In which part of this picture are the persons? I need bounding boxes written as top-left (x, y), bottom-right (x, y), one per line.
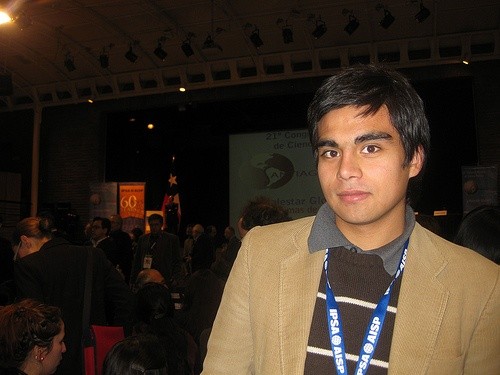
top-left (198, 62), bottom-right (500, 375)
top-left (0, 192), bottom-right (500, 375)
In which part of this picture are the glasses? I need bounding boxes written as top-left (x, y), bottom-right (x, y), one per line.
top-left (13, 241), bottom-right (22, 260)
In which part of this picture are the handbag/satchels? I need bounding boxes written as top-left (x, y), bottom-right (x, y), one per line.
top-left (82, 325), bottom-right (125, 375)
